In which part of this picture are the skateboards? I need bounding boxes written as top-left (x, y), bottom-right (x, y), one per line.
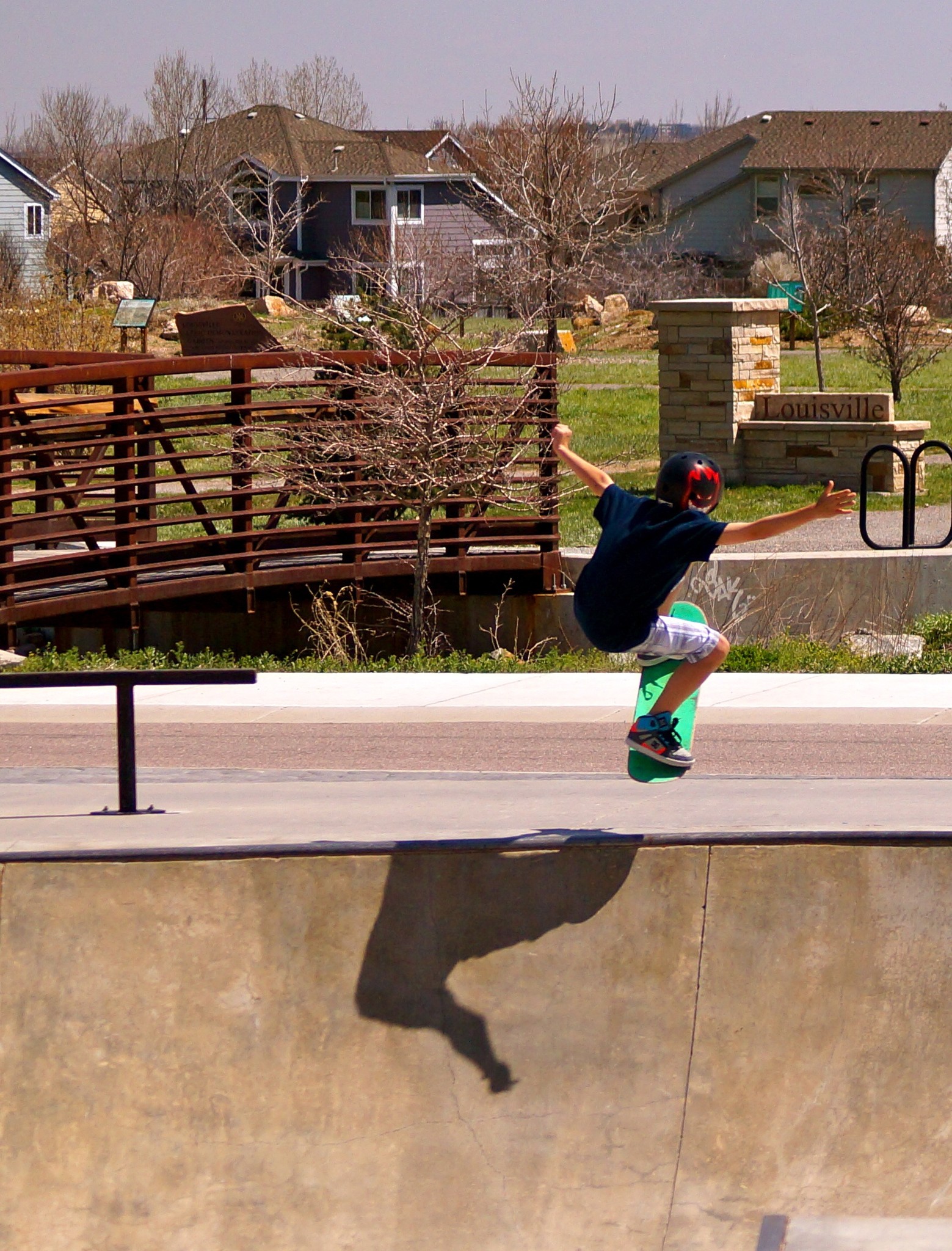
top-left (627, 601), bottom-right (708, 783)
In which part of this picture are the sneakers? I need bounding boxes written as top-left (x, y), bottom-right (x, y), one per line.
top-left (626, 711), bottom-right (697, 766)
top-left (636, 654), bottom-right (669, 666)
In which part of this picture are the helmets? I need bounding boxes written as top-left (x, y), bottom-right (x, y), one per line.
top-left (655, 451), bottom-right (723, 515)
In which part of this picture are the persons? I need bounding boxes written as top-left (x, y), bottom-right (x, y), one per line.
top-left (551, 423), bottom-right (857, 767)
